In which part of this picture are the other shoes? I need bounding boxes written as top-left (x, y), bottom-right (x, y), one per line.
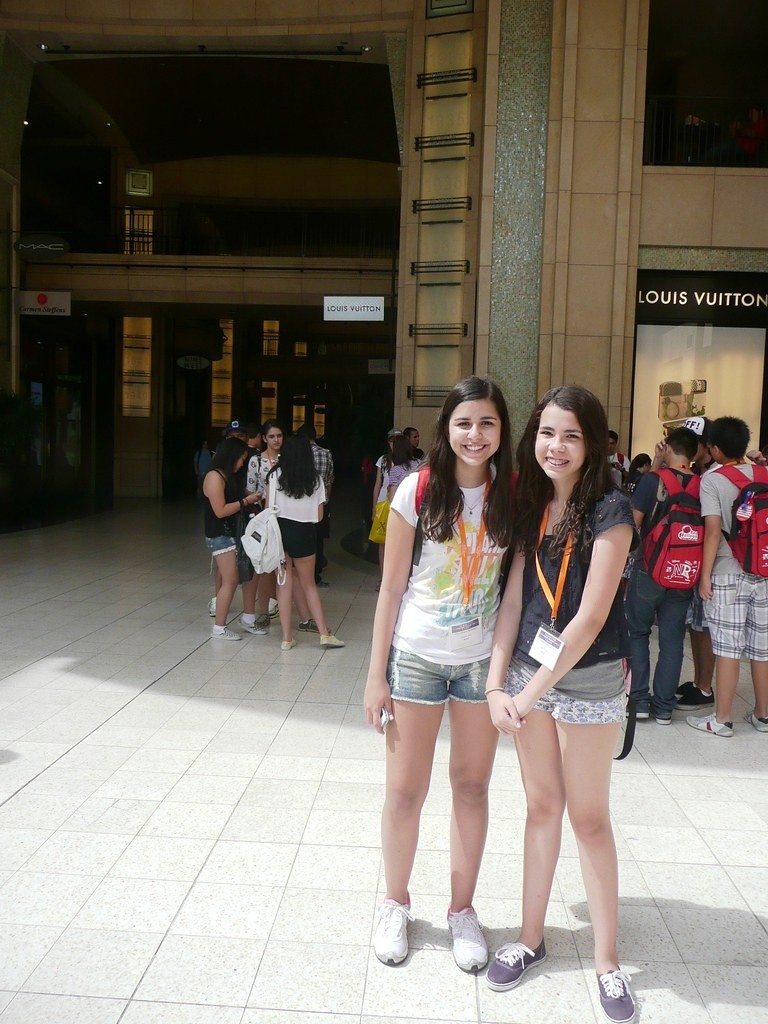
top-left (238, 615), bottom-right (268, 635)
top-left (257, 614), bottom-right (271, 626)
top-left (269, 607), bottom-right (279, 618)
top-left (210, 627), bottom-right (241, 640)
top-left (208, 600), bottom-right (217, 617)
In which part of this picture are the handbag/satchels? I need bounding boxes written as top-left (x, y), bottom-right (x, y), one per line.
top-left (236, 499), bottom-right (255, 583)
top-left (368, 500), bottom-right (390, 544)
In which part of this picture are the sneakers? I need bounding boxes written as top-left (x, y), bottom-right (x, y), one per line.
top-left (650, 707), bottom-right (671, 724)
top-left (374, 890), bottom-right (414, 965)
top-left (446, 900), bottom-right (488, 973)
top-left (674, 687), bottom-right (715, 710)
top-left (744, 710), bottom-right (768, 732)
top-left (626, 702), bottom-right (649, 718)
top-left (486, 936), bottom-right (546, 992)
top-left (298, 619), bottom-right (331, 633)
top-left (674, 681), bottom-right (696, 698)
top-left (319, 635), bottom-right (344, 648)
top-left (686, 713), bottom-right (734, 737)
top-left (281, 637), bottom-right (296, 651)
top-left (596, 964), bottom-right (637, 1024)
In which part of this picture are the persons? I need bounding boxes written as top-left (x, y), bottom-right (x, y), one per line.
top-left (403, 427), bottom-right (420, 447)
top-left (626, 427), bottom-right (698, 725)
top-left (674, 416), bottom-right (719, 711)
top-left (608, 431), bottom-right (631, 489)
top-left (369, 428), bottom-right (402, 592)
top-left (194, 419), bottom-right (286, 635)
top-left (362, 376), bottom-right (519, 971)
top-left (686, 416), bottom-right (768, 737)
top-left (265, 431), bottom-right (345, 651)
top-left (486, 385), bottom-right (639, 1024)
top-left (625, 453), bottom-right (652, 498)
top-left (389, 435), bottom-right (422, 502)
top-left (201, 437), bottom-right (263, 641)
top-left (296, 423), bottom-right (334, 587)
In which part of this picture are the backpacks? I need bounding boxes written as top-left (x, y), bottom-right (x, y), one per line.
top-left (709, 464), bottom-right (768, 577)
top-left (643, 469), bottom-right (703, 589)
top-left (241, 471), bottom-right (286, 586)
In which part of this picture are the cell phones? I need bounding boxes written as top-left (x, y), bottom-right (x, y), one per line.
top-left (380, 706), bottom-right (389, 729)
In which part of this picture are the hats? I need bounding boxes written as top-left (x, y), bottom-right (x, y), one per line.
top-left (226, 421), bottom-right (247, 433)
top-left (683, 417), bottom-right (713, 437)
top-left (388, 428), bottom-right (402, 439)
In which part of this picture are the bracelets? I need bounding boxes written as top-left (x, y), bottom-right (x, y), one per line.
top-left (245, 498), bottom-right (249, 506)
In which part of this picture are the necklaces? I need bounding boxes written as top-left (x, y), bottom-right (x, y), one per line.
top-left (461, 497), bottom-right (483, 515)
top-left (554, 498), bottom-right (568, 520)
